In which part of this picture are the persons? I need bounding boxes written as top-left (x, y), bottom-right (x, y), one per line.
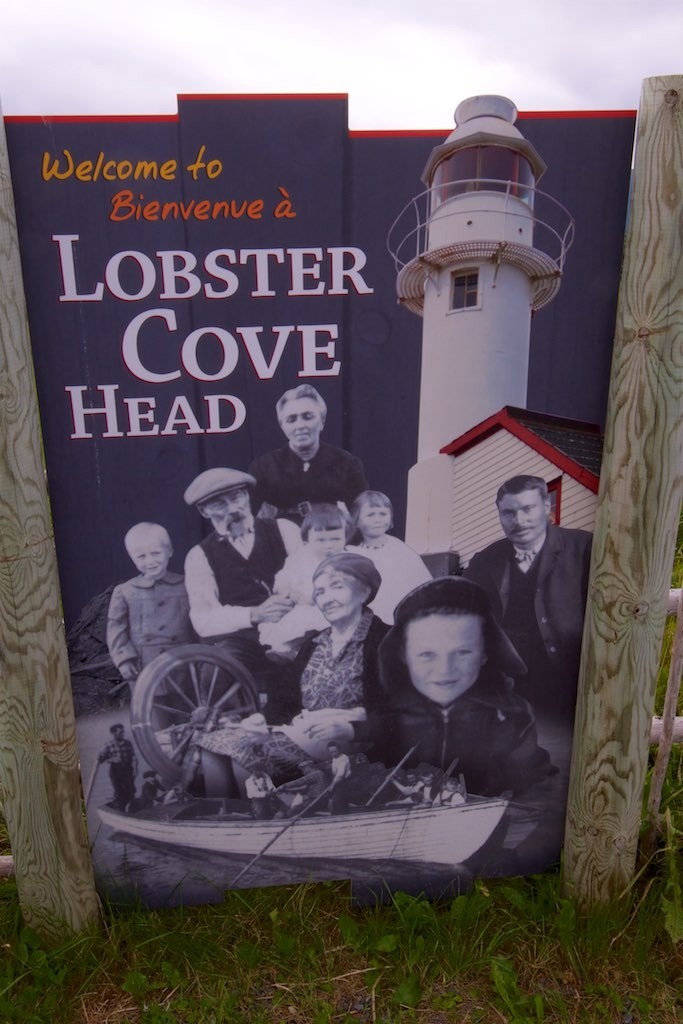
top-left (91, 382), bottom-right (595, 822)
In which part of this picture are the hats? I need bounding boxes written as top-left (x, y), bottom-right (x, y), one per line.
top-left (376, 575), bottom-right (528, 680)
top-left (182, 468), bottom-right (255, 507)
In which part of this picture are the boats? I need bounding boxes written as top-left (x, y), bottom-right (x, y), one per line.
top-left (94, 779), bottom-right (513, 869)
